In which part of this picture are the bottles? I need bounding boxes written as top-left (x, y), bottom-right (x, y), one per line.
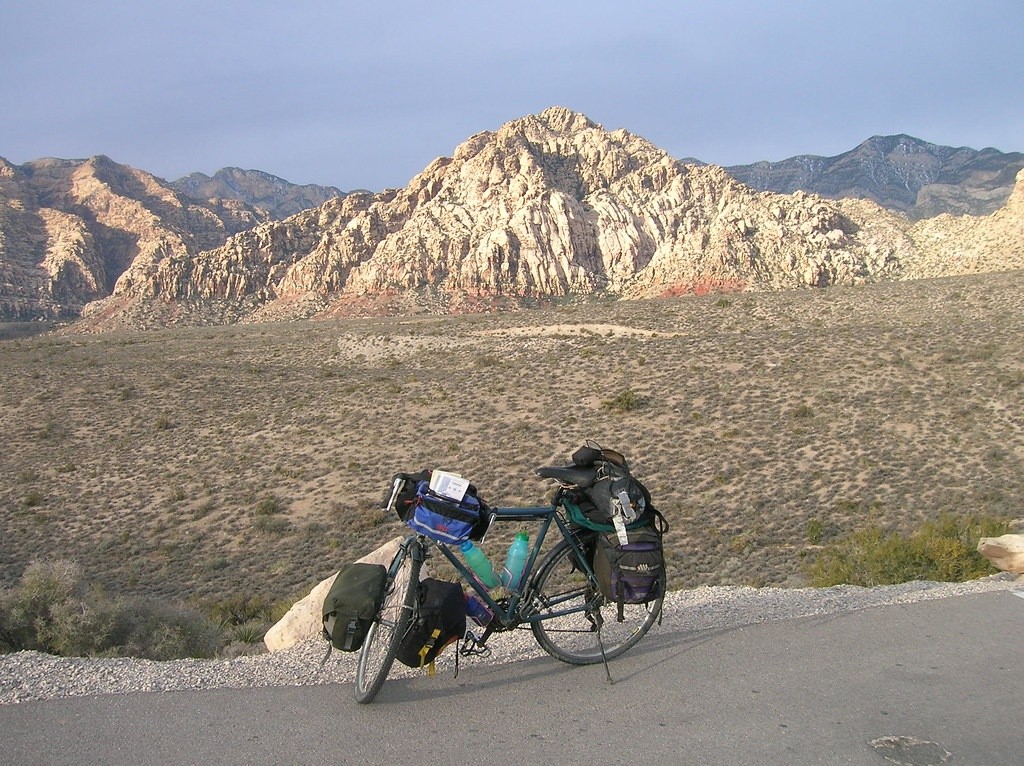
top-left (466, 596), bottom-right (493, 627)
top-left (460, 540), bottom-right (502, 589)
top-left (501, 530), bottom-right (529, 591)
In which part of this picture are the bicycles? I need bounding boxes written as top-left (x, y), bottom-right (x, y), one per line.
top-left (354, 463), bottom-right (667, 703)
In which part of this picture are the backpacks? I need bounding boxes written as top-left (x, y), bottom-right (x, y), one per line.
top-left (395, 577), bottom-right (466, 680)
top-left (319, 563), bottom-right (387, 664)
top-left (593, 526), bottom-right (666, 626)
top-left (562, 448), bottom-right (654, 533)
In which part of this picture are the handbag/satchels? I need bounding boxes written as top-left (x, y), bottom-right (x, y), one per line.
top-left (397, 469), bottom-right (497, 545)
